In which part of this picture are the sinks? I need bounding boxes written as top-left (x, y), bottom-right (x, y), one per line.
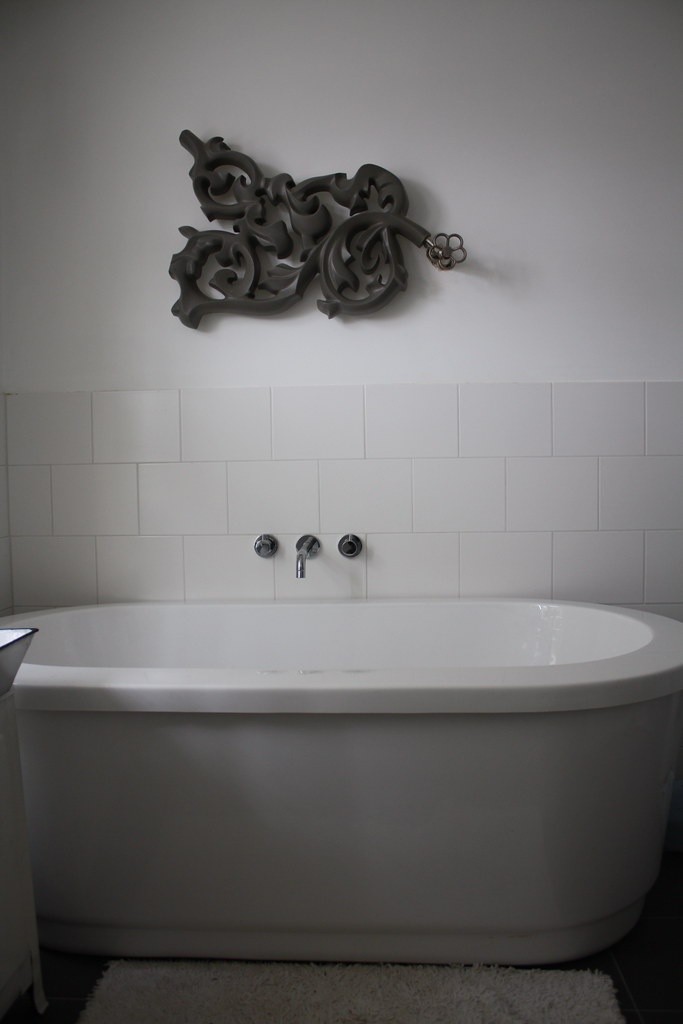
top-left (0, 629), bottom-right (39, 700)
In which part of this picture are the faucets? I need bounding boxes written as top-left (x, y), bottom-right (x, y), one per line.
top-left (296, 537), bottom-right (315, 579)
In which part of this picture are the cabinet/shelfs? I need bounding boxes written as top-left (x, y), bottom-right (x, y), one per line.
top-left (0, 691), bottom-right (48, 1015)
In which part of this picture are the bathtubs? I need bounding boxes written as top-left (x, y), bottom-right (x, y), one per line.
top-left (0, 602), bottom-right (682, 964)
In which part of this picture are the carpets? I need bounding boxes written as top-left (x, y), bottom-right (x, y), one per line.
top-left (80, 960), bottom-right (626, 1024)
